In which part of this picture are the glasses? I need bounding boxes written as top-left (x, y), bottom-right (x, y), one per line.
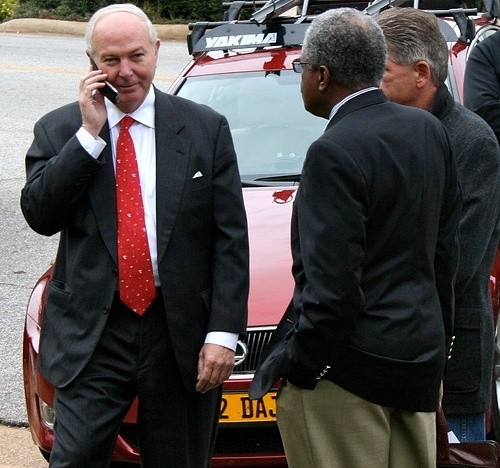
top-left (291, 58), bottom-right (311, 72)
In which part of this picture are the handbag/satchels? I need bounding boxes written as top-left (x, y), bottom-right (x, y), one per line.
top-left (438, 404), bottom-right (497, 468)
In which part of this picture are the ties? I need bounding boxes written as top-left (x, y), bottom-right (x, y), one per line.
top-left (114, 116), bottom-right (156, 317)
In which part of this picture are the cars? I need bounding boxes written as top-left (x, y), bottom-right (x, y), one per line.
top-left (21, 0), bottom-right (500, 468)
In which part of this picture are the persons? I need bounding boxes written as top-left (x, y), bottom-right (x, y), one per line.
top-left (247, 8), bottom-right (463, 468)
top-left (463, 28), bottom-right (500, 148)
top-left (371, 7), bottom-right (500, 442)
top-left (20, 3), bottom-right (249, 468)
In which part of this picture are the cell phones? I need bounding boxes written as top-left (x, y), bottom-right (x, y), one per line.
top-left (90, 58), bottom-right (118, 104)
